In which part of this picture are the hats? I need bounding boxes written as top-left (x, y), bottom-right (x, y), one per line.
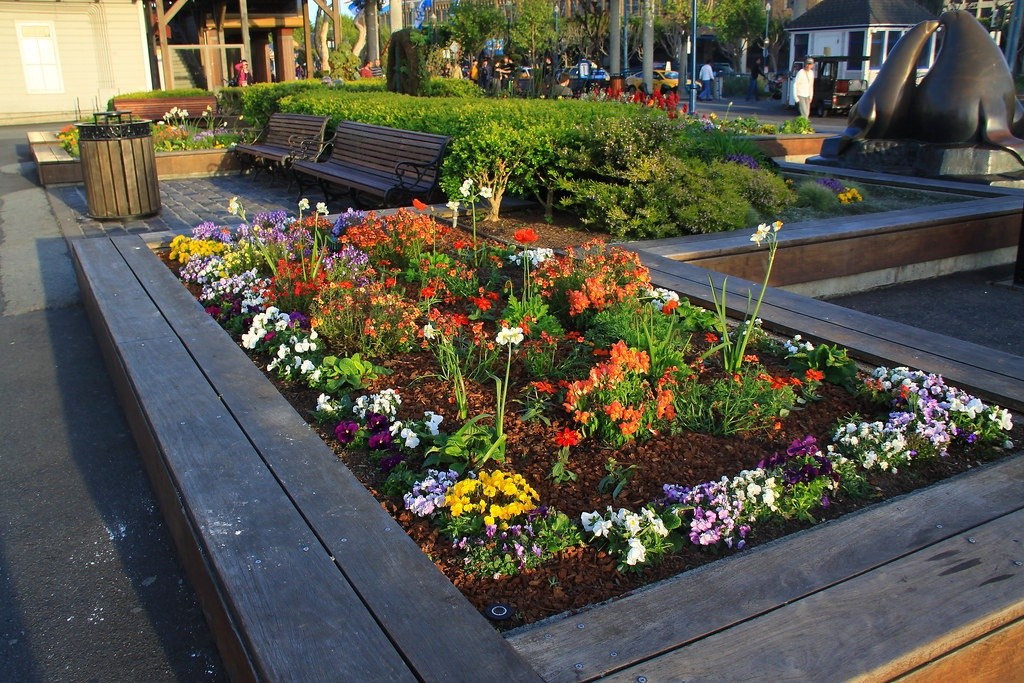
top-left (805, 58), bottom-right (814, 64)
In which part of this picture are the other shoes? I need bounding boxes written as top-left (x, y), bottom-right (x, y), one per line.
top-left (698, 96), bottom-right (702, 101)
top-left (706, 99), bottom-right (712, 101)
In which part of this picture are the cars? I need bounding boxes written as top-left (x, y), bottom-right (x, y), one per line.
top-left (623, 68), bottom-right (705, 101)
top-left (620, 61), bottom-right (790, 100)
top-left (459, 56), bottom-right (611, 99)
top-left (805, 54), bottom-right (873, 119)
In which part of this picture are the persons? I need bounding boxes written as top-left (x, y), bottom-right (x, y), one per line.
top-left (698, 60), bottom-right (714, 101)
top-left (492, 56), bottom-right (516, 94)
top-left (551, 76), bottom-right (573, 96)
top-left (793, 58), bottom-right (814, 119)
top-left (371, 59), bottom-right (382, 76)
top-left (296, 66), bottom-right (301, 77)
top-left (542, 58), bottom-right (554, 83)
top-left (362, 61), bottom-right (373, 78)
top-left (440, 61), bottom-right (462, 79)
top-left (235, 60), bottom-right (252, 86)
top-left (462, 64), bottom-right (468, 77)
top-left (477, 58), bottom-right (492, 92)
top-left (575, 56), bottom-right (597, 93)
top-left (469, 60), bottom-right (479, 80)
top-left (746, 58), bottom-right (766, 101)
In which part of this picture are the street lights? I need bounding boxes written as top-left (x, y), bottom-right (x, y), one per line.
top-left (553, 2), bottom-right (561, 62)
top-left (763, 3), bottom-right (772, 80)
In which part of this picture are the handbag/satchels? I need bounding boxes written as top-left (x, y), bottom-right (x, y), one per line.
top-left (230, 77), bottom-right (237, 86)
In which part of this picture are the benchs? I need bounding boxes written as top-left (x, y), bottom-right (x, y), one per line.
top-left (114, 96), bottom-right (227, 130)
top-left (234, 111), bottom-right (451, 211)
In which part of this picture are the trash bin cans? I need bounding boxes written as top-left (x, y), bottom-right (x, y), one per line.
top-left (74, 110), bottom-right (162, 219)
top-left (610, 76), bottom-right (625, 96)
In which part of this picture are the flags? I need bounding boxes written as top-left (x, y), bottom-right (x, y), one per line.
top-left (380, 3), bottom-right (390, 13)
top-left (415, 0), bottom-right (431, 29)
top-left (348, 2), bottom-right (360, 15)
